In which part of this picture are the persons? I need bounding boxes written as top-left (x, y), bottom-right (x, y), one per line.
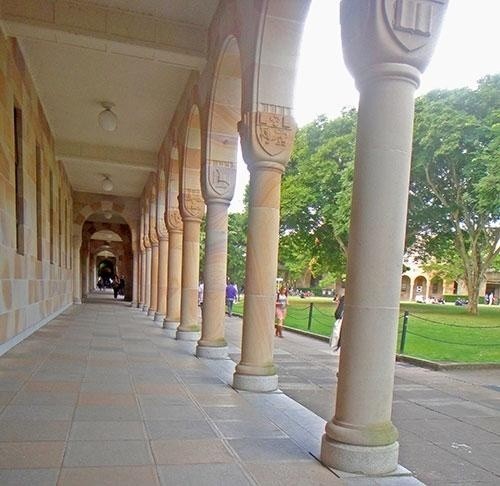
top-left (486, 292), bottom-right (495, 305)
top-left (97, 275), bottom-right (126, 300)
top-left (226, 281), bottom-right (237, 317)
top-left (288, 287), bottom-right (312, 298)
top-left (431, 297), bottom-right (469, 306)
top-left (198, 283), bottom-right (204, 316)
top-left (329, 296), bottom-right (345, 351)
top-left (275, 287), bottom-right (289, 339)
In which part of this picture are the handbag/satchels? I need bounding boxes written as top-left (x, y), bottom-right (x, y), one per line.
top-left (330, 319), bottom-right (343, 348)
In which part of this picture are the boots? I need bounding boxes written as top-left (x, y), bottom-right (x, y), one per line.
top-left (278, 326), bottom-right (284, 337)
top-left (276, 326), bottom-right (278, 336)
